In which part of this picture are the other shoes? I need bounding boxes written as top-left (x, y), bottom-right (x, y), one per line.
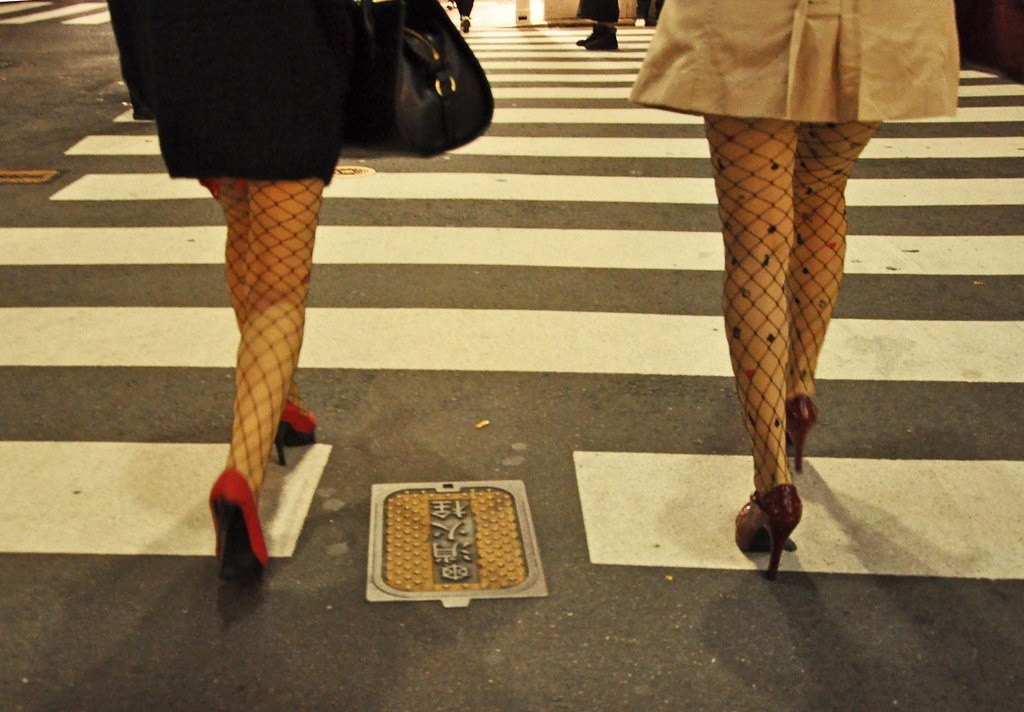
top-left (576, 24), bottom-right (618, 50)
top-left (461, 16), bottom-right (470, 33)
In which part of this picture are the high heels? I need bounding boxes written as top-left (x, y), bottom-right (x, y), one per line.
top-left (275, 399), bottom-right (318, 466)
top-left (735, 484), bottom-right (803, 578)
top-left (208, 469), bottom-right (270, 591)
top-left (786, 393), bottom-right (820, 475)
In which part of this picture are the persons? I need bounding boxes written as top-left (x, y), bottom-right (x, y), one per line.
top-left (575, 0), bottom-right (620, 50)
top-left (630, 0), bottom-right (960, 579)
top-left (446, 0), bottom-right (474, 33)
top-left (635, 0), bottom-right (665, 27)
top-left (108, 0), bottom-right (367, 584)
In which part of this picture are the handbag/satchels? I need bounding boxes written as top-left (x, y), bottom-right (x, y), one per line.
top-left (360, 0), bottom-right (494, 156)
